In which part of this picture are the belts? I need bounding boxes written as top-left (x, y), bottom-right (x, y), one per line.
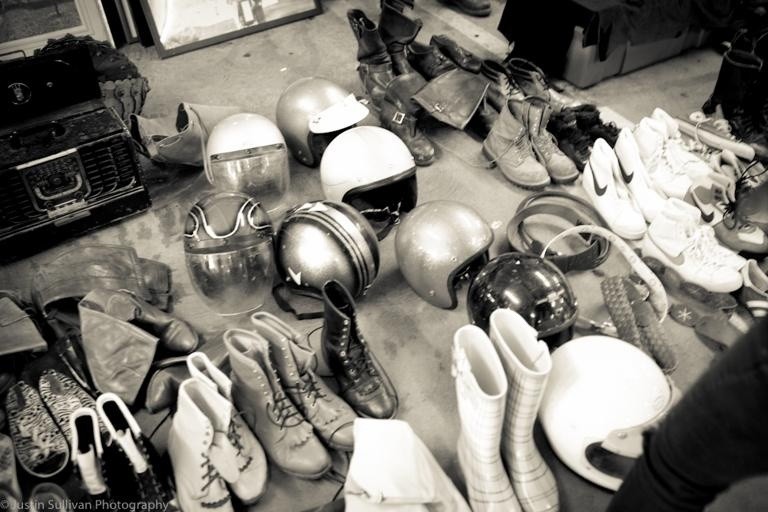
top-left (506, 190), bottom-right (612, 271)
top-left (601, 276), bottom-right (680, 374)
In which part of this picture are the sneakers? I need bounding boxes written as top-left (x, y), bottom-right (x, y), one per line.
top-left (580, 107), bottom-right (768, 323)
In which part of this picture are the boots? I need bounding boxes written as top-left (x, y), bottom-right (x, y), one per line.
top-left (450, 324), bottom-right (523, 512)
top-left (0, 245), bottom-right (398, 512)
top-left (343, 416), bottom-right (471, 510)
top-left (701, 50), bottom-right (767, 147)
top-left (346, 0), bottom-right (621, 192)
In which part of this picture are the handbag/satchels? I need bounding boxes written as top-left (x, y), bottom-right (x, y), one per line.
top-left (128, 100), bottom-right (240, 170)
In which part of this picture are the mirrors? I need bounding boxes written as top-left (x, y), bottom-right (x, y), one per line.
top-left (138, 0), bottom-right (325, 61)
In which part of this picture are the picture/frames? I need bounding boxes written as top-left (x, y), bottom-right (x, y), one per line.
top-left (0, 0), bottom-right (117, 62)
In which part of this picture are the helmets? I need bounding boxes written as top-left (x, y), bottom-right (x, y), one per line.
top-left (538, 336), bottom-right (683, 496)
top-left (488, 308), bottom-right (561, 512)
top-left (183, 76), bottom-right (419, 318)
top-left (394, 198), bottom-right (494, 312)
top-left (468, 252), bottom-right (578, 347)
